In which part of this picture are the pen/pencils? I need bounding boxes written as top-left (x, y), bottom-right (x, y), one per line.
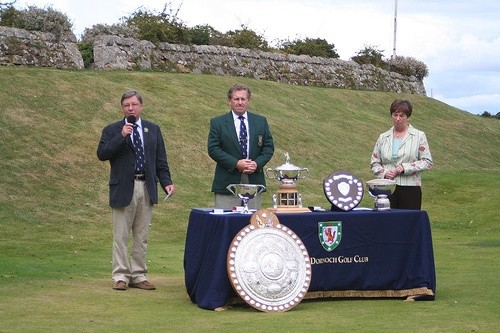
top-left (164, 194), bottom-right (169, 201)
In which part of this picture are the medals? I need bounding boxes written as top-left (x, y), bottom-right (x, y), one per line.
top-left (227, 210), bottom-right (312, 313)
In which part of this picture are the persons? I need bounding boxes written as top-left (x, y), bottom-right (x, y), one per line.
top-left (96, 91), bottom-right (175, 291)
top-left (369, 99), bottom-right (434, 210)
top-left (206, 83), bottom-right (276, 210)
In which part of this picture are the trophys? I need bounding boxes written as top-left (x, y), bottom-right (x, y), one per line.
top-left (323, 168), bottom-right (364, 212)
top-left (365, 179), bottom-right (398, 211)
top-left (226, 183), bottom-right (267, 214)
top-left (265, 152), bottom-right (311, 213)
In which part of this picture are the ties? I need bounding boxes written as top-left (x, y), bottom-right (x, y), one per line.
top-left (237, 115), bottom-right (247, 161)
top-left (132, 124), bottom-right (145, 173)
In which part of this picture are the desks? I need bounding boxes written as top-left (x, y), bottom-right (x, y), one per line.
top-left (184, 208), bottom-right (436, 311)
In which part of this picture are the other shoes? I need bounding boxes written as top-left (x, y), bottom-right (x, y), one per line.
top-left (111, 281), bottom-right (129, 289)
top-left (130, 280), bottom-right (157, 289)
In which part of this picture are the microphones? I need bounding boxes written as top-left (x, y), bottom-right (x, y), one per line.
top-left (125, 115), bottom-right (136, 145)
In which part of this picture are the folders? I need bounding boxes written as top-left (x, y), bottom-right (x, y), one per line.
top-left (191, 208), bottom-right (233, 213)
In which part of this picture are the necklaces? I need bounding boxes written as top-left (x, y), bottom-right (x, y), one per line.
top-left (394, 129), bottom-right (407, 137)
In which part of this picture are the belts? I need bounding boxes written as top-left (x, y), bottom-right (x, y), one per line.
top-left (134, 176), bottom-right (144, 181)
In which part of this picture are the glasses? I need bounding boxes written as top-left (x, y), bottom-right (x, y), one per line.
top-left (122, 102), bottom-right (140, 108)
